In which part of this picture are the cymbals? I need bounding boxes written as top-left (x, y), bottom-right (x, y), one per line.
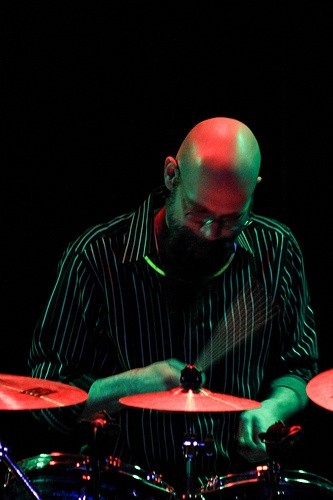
top-left (0, 371), bottom-right (89, 412)
top-left (305, 368), bottom-right (333, 413)
top-left (118, 365), bottom-right (263, 413)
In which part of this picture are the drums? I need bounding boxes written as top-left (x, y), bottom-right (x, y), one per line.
top-left (0, 450), bottom-right (174, 498)
top-left (193, 461), bottom-right (333, 499)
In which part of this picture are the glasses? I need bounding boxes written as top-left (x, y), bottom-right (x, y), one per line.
top-left (178, 167), bottom-right (255, 232)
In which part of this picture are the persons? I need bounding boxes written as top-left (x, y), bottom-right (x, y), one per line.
top-left (27, 117), bottom-right (319, 464)
